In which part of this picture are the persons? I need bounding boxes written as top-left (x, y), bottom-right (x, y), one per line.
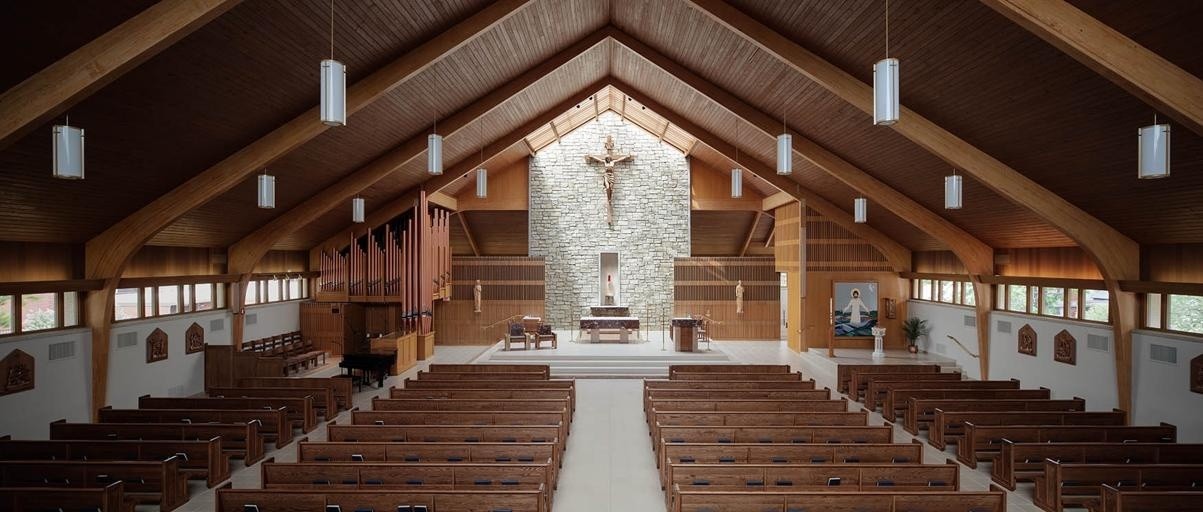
top-left (842, 288), bottom-right (871, 324)
top-left (735, 279), bottom-right (745, 312)
top-left (589, 155), bottom-right (631, 203)
top-left (474, 280), bottom-right (481, 311)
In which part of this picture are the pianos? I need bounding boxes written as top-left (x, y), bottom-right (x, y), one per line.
top-left (339, 347), bottom-right (398, 391)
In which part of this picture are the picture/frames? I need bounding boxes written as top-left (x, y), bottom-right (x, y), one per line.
top-left (832, 278), bottom-right (880, 340)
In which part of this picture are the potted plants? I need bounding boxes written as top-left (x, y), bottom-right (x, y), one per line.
top-left (903, 316), bottom-right (929, 353)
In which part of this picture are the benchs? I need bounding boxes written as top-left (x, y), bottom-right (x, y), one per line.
top-left (1, 329), bottom-right (573, 511)
top-left (642, 361), bottom-right (1203, 509)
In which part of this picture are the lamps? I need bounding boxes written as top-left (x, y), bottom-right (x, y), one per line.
top-left (427, 63), bottom-right (443, 174)
top-left (321, 0), bottom-right (348, 127)
top-left (352, 194), bottom-right (366, 223)
top-left (732, 116), bottom-right (744, 199)
top-left (777, 61), bottom-right (793, 176)
top-left (945, 165), bottom-right (962, 209)
top-left (855, 194), bottom-right (867, 223)
top-left (477, 118), bottom-right (488, 197)
top-left (872, 0), bottom-right (901, 126)
top-left (52, 113), bottom-right (86, 181)
top-left (1137, 115), bottom-right (1177, 181)
top-left (258, 168), bottom-right (277, 208)
